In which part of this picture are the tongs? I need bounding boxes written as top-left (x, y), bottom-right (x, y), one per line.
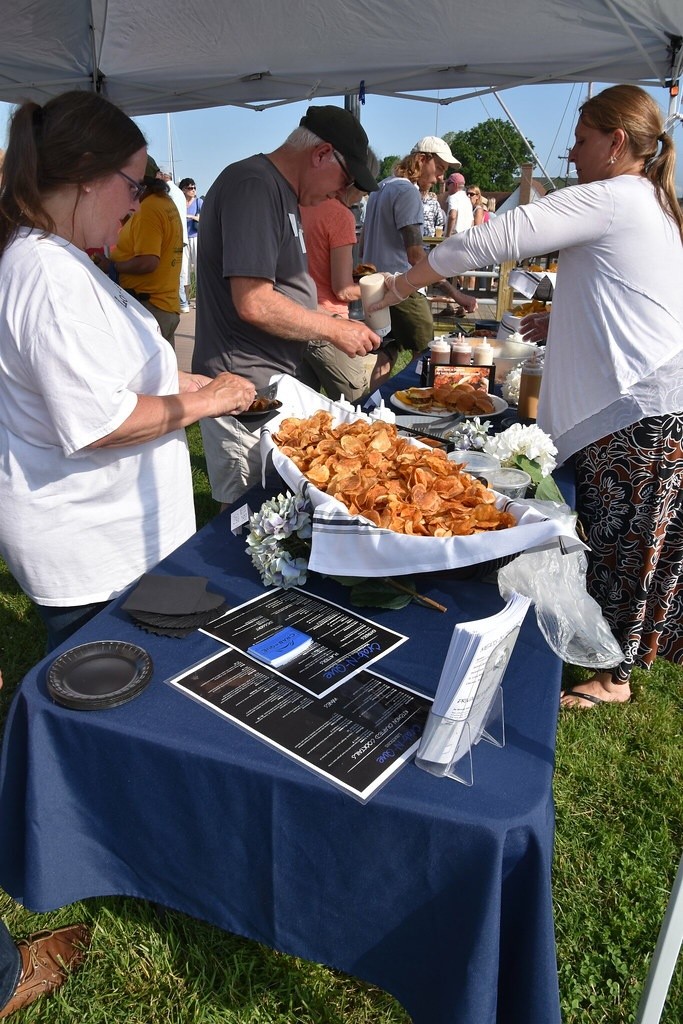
top-left (412, 413), bottom-right (464, 438)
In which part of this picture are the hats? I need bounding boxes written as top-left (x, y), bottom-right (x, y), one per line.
top-left (301, 106), bottom-right (380, 193)
top-left (444, 173), bottom-right (465, 185)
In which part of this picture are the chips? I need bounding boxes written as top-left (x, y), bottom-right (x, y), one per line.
top-left (272, 409), bottom-right (517, 537)
top-left (513, 299), bottom-right (552, 315)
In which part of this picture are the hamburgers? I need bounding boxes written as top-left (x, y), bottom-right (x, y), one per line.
top-left (396, 382), bottom-right (495, 415)
top-left (359, 261), bottom-right (376, 275)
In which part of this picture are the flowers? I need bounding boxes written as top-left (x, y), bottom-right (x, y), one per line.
top-left (449, 418), bottom-right (589, 539)
top-left (244, 491), bottom-right (447, 610)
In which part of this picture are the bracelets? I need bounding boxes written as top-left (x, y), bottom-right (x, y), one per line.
top-left (405, 272), bottom-right (419, 290)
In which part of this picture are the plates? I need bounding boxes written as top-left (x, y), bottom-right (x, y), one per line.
top-left (48, 640), bottom-right (154, 711)
top-left (234, 399), bottom-right (282, 417)
top-left (389, 387), bottom-right (508, 418)
top-left (392, 416), bottom-right (466, 451)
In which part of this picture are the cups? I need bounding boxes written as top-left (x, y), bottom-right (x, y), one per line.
top-left (435, 225), bottom-right (444, 237)
top-left (446, 450), bottom-right (530, 499)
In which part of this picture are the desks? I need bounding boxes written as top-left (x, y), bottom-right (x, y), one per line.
top-left (0, 349), bottom-right (573, 1024)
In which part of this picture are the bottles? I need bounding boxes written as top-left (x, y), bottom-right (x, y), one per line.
top-left (430, 332), bottom-right (493, 368)
top-left (334, 392), bottom-right (395, 425)
top-left (523, 250), bottom-right (559, 271)
top-left (359, 273), bottom-right (392, 337)
top-left (518, 351), bottom-right (543, 419)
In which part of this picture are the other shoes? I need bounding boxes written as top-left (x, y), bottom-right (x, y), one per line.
top-left (180, 307), bottom-right (189, 312)
top-left (0, 922), bottom-right (90, 1018)
top-left (454, 309), bottom-right (467, 316)
top-left (434, 308), bottom-right (454, 317)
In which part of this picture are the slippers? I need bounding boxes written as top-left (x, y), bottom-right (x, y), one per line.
top-left (559, 685), bottom-right (635, 713)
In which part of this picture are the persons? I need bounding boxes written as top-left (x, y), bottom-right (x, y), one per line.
top-left (0, 91), bottom-right (255, 648)
top-left (420, 172), bottom-right (497, 317)
top-left (299, 145), bottom-right (380, 406)
top-left (0, 918), bottom-right (91, 1019)
top-left (190, 105), bottom-right (382, 511)
top-left (368, 85), bottom-right (683, 708)
top-left (156, 169), bottom-right (189, 314)
top-left (358, 136), bottom-right (479, 394)
top-left (93, 154), bottom-right (183, 348)
top-left (178, 178), bottom-right (203, 308)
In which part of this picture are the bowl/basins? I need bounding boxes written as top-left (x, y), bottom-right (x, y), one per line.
top-left (475, 319), bottom-right (499, 332)
top-left (427, 337), bottom-right (545, 378)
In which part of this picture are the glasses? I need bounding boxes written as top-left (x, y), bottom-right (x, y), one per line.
top-left (185, 187), bottom-right (195, 191)
top-left (96, 154), bottom-right (148, 202)
top-left (447, 181), bottom-right (454, 185)
top-left (410, 136), bottom-right (462, 170)
top-left (313, 143), bottom-right (355, 189)
top-left (466, 192), bottom-right (478, 197)
